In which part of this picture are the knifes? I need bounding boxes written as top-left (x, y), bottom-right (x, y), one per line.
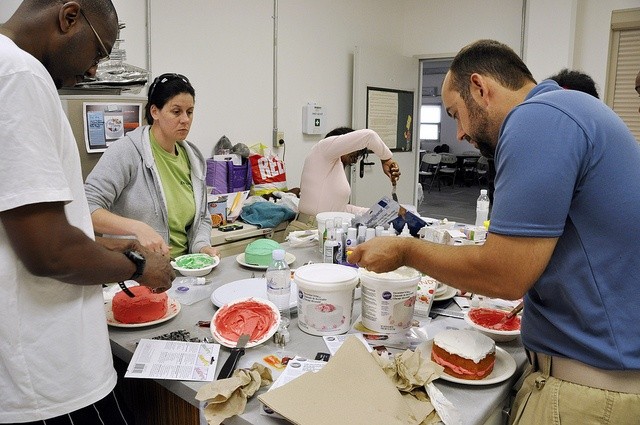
top-left (216, 314), bottom-right (261, 386)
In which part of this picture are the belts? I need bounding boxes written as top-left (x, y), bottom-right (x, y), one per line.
top-left (525, 347), bottom-right (640, 395)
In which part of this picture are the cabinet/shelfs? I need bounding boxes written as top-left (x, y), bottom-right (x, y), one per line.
top-left (457, 151), bottom-right (480, 185)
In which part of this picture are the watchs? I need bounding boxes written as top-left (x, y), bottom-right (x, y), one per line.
top-left (118, 249), bottom-right (146, 298)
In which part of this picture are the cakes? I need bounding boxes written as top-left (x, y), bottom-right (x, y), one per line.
top-left (244, 239), bottom-right (281, 267)
top-left (431, 329), bottom-right (496, 381)
top-left (111, 285), bottom-right (169, 324)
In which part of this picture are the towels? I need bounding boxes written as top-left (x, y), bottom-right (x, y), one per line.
top-left (286, 229), bottom-right (318, 245)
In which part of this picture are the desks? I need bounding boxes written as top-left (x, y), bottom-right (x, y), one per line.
top-left (456, 155), bottom-right (481, 188)
top-left (105, 241), bottom-right (530, 425)
top-left (211, 223), bottom-right (288, 257)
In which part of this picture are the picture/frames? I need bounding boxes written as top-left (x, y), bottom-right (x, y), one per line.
top-left (366, 86), bottom-right (414, 152)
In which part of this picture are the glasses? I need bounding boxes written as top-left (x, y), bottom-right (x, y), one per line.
top-left (150, 72), bottom-right (189, 101)
top-left (62, 0), bottom-right (110, 64)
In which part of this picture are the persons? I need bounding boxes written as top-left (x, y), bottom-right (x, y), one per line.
top-left (283, 126), bottom-right (401, 242)
top-left (0, 0), bottom-right (177, 425)
top-left (345, 38), bottom-right (640, 425)
top-left (549, 67), bottom-right (600, 100)
top-left (83, 72), bottom-right (221, 263)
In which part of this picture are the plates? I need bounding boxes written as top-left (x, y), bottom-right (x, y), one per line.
top-left (236, 251), bottom-right (299, 268)
top-left (208, 297), bottom-right (282, 350)
top-left (208, 276), bottom-right (315, 312)
top-left (101, 285), bottom-right (180, 328)
top-left (414, 336), bottom-right (518, 387)
top-left (433, 280), bottom-right (459, 303)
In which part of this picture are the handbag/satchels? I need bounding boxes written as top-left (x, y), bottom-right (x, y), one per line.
top-left (206, 156), bottom-right (252, 194)
top-left (247, 142), bottom-right (287, 197)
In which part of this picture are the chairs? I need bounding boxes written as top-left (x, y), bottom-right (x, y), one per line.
top-left (439, 153), bottom-right (458, 191)
top-left (419, 154), bottom-right (441, 194)
top-left (478, 157), bottom-right (489, 189)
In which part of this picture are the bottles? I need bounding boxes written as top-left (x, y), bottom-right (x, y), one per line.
top-left (323, 216), bottom-right (415, 266)
top-left (265, 247), bottom-right (292, 330)
top-left (474, 188), bottom-right (490, 242)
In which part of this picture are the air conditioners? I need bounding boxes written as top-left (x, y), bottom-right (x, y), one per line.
top-left (422, 87), bottom-right (437, 98)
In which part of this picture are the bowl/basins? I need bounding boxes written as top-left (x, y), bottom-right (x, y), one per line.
top-left (171, 253), bottom-right (221, 278)
top-left (465, 306), bottom-right (526, 343)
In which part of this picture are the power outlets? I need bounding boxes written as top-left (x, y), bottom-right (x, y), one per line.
top-left (274, 129), bottom-right (284, 148)
